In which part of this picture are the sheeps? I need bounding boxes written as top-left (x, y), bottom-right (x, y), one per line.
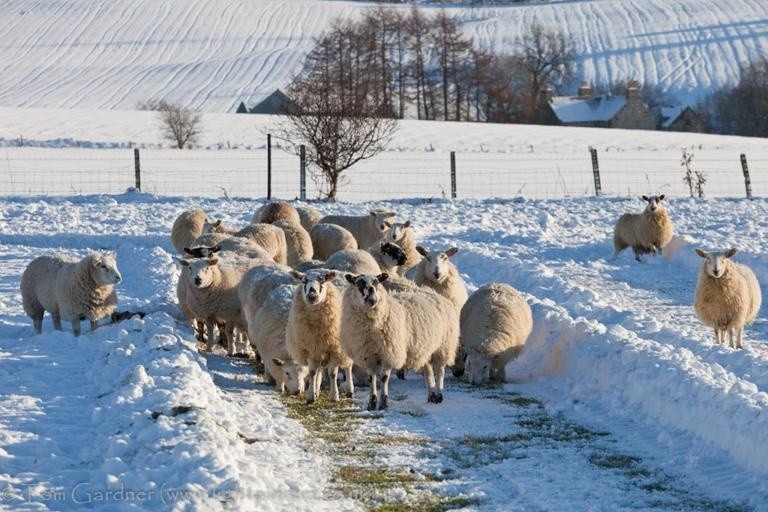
top-left (614, 194), bottom-right (673, 262)
top-left (171, 199), bottom-right (468, 412)
top-left (693, 247), bottom-right (762, 349)
top-left (20, 250), bottom-right (123, 338)
top-left (460, 282), bottom-right (533, 386)
